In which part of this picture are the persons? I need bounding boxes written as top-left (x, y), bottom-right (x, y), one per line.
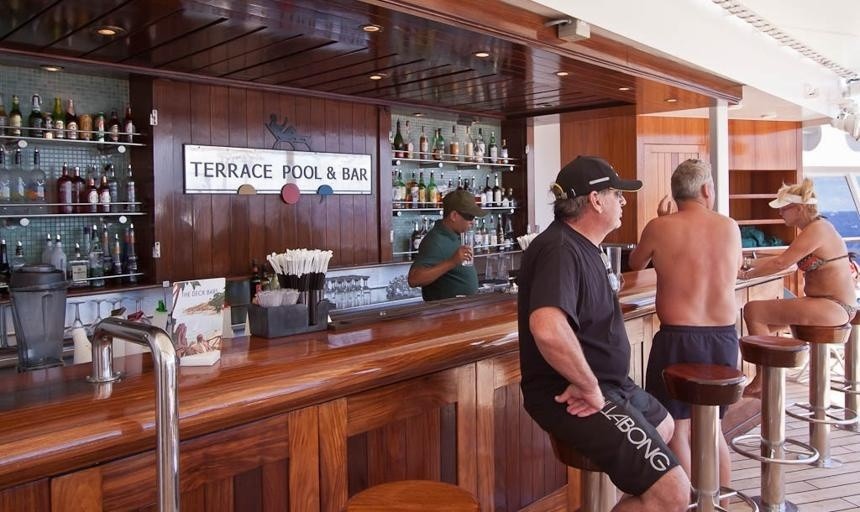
top-left (518, 155), bottom-right (692, 512)
top-left (408, 189), bottom-right (488, 301)
top-left (629, 159), bottom-right (742, 512)
top-left (737, 177), bottom-right (857, 394)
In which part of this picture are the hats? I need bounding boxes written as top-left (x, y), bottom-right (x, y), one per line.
top-left (552, 157), bottom-right (642, 193)
top-left (443, 190), bottom-right (488, 217)
top-left (768, 191), bottom-right (817, 209)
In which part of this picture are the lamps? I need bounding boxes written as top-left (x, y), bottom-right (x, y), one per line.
top-left (359, 22), bottom-right (384, 34)
top-left (95, 25), bottom-right (125, 40)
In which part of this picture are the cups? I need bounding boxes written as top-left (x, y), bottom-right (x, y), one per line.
top-left (325, 275), bottom-right (370, 299)
top-left (108, 299), bottom-right (118, 310)
top-left (117, 297), bottom-right (128, 308)
top-left (461, 232), bottom-right (473, 266)
top-left (132, 298), bottom-right (140, 312)
top-left (90, 300), bottom-right (105, 327)
top-left (607, 246), bottom-right (621, 292)
top-left (68, 302), bottom-right (86, 327)
top-left (138, 295), bottom-right (146, 310)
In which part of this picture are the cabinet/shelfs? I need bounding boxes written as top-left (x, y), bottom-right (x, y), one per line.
top-left (729, 119), bottom-right (804, 247)
top-left (625, 315), bottom-right (654, 391)
top-left (50, 406), bottom-right (320, 512)
top-left (478, 352), bottom-right (581, 511)
top-left (0, 125), bottom-right (150, 286)
top-left (749, 279), bottom-right (787, 430)
top-left (561, 116), bottom-right (710, 245)
top-left (319, 363), bottom-right (478, 512)
top-left (733, 288), bottom-right (749, 437)
top-left (392, 147), bottom-right (523, 256)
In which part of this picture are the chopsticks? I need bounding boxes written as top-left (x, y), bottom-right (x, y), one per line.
top-left (268, 249), bottom-right (333, 291)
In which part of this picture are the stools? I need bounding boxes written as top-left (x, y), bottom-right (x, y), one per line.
top-left (551, 436), bottom-right (619, 512)
top-left (791, 321), bottom-right (852, 469)
top-left (831, 311), bottom-right (860, 432)
top-left (342, 479), bottom-right (479, 511)
top-left (662, 363), bottom-right (761, 511)
top-left (739, 336), bottom-right (812, 511)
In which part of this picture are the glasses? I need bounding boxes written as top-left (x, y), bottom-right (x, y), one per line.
top-left (456, 211), bottom-right (475, 220)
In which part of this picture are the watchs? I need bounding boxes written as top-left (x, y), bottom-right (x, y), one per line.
top-left (747, 257), bottom-right (754, 269)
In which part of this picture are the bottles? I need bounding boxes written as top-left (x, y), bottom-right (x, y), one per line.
top-left (29, 147), bottom-right (48, 214)
top-left (0, 150), bottom-right (13, 216)
top-left (475, 127), bottom-right (486, 165)
top-left (93, 112), bottom-right (108, 141)
top-left (474, 217), bottom-right (483, 256)
top-left (474, 186), bottom-right (486, 208)
top-left (107, 164), bottom-right (123, 213)
top-left (65, 99), bottom-right (80, 139)
top-left (464, 178), bottom-right (471, 191)
top-left (28, 93), bottom-right (44, 138)
top-left (464, 126), bottom-right (474, 162)
top-left (1, 92), bottom-right (9, 136)
top-left (481, 218), bottom-right (490, 254)
top-left (408, 171), bottom-right (419, 210)
top-left (6, 95), bottom-right (23, 137)
top-left (394, 119), bottom-right (406, 159)
top-left (412, 221), bottom-right (422, 260)
top-left (83, 177), bottom-right (99, 213)
top-left (392, 168), bottom-right (402, 209)
top-left (40, 233), bottom-right (55, 265)
top-left (57, 161), bottom-right (74, 214)
top-left (122, 164), bottom-right (140, 212)
top-left (68, 243), bottom-right (90, 289)
top-left (448, 178), bottom-right (455, 193)
top-left (108, 233), bottom-right (125, 286)
top-left (98, 225), bottom-right (115, 278)
top-left (106, 110), bottom-right (123, 141)
top-left (495, 214), bottom-right (505, 252)
top-left (42, 111), bottom-right (55, 138)
top-left (488, 131), bottom-right (499, 164)
top-left (10, 242), bottom-right (29, 274)
top-left (488, 215), bottom-right (498, 253)
top-left (418, 172), bottom-right (427, 208)
top-left (500, 140), bottom-right (510, 164)
top-left (422, 217), bottom-right (429, 238)
top-left (121, 102), bottom-right (136, 143)
top-left (79, 114), bottom-right (94, 141)
top-left (507, 188), bottom-right (518, 207)
top-left (250, 258), bottom-right (262, 302)
top-left (493, 173), bottom-right (502, 207)
top-left (467, 177), bottom-right (477, 195)
top-left (122, 223), bottom-right (140, 284)
top-left (449, 125), bottom-right (460, 162)
top-left (72, 166), bottom-right (86, 214)
top-left (261, 264), bottom-right (272, 293)
top-left (456, 176), bottom-right (464, 190)
top-left (436, 129), bottom-right (445, 159)
top-left (399, 169), bottom-right (407, 209)
top-left (98, 176), bottom-right (112, 213)
top-left (50, 234), bottom-right (67, 282)
top-left (406, 122), bottom-right (414, 160)
top-left (431, 130), bottom-right (438, 157)
top-left (82, 227), bottom-right (91, 256)
top-left (53, 96), bottom-right (67, 140)
top-left (86, 224), bottom-right (106, 288)
top-left (427, 170), bottom-right (438, 208)
top-left (501, 187), bottom-right (510, 206)
top-left (9, 148), bottom-right (30, 215)
top-left (123, 227), bottom-right (130, 270)
top-left (271, 271), bottom-right (280, 290)
top-left (1, 238), bottom-right (11, 297)
top-left (419, 126), bottom-right (428, 160)
top-left (507, 219), bottom-right (515, 251)
top-left (437, 172), bottom-right (448, 208)
top-left (483, 174), bottom-right (494, 209)
top-left (503, 228), bottom-right (510, 252)
